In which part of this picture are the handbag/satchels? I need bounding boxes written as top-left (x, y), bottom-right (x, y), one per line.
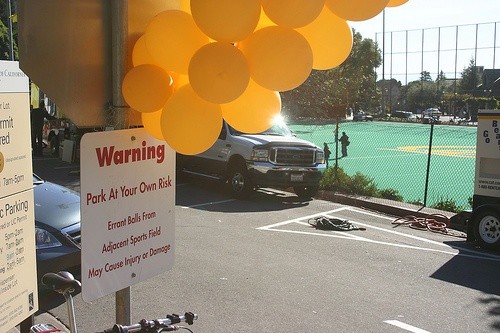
top-left (346, 141), bottom-right (350, 146)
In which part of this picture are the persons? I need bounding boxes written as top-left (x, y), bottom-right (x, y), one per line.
top-left (31, 103), bottom-right (55, 155)
top-left (339, 132), bottom-right (349, 157)
top-left (324, 143), bottom-right (330, 163)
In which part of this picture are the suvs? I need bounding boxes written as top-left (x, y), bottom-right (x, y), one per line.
top-left (176, 114), bottom-right (327, 201)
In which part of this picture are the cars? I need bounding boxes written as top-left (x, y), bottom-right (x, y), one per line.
top-left (354, 109), bottom-right (374, 122)
top-left (33, 173), bottom-right (81, 281)
top-left (422, 107), bottom-right (442, 119)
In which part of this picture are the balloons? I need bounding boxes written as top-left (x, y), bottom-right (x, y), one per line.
top-left (160, 84), bottom-right (222, 155)
top-left (142, 108), bottom-right (164, 140)
top-left (221, 79), bottom-right (281, 133)
top-left (145, 10), bottom-right (208, 75)
top-left (189, 42), bottom-right (250, 103)
top-left (254, 0), bottom-right (409, 70)
top-left (132, 35), bottom-right (188, 88)
top-left (190, 0), bottom-right (261, 42)
top-left (122, 64), bottom-right (174, 113)
top-left (239, 26), bottom-right (313, 92)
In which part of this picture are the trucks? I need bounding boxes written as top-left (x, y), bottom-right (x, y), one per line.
top-left (466, 106), bottom-right (500, 254)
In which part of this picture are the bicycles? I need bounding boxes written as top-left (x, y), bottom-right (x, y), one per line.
top-left (28, 271), bottom-right (199, 333)
